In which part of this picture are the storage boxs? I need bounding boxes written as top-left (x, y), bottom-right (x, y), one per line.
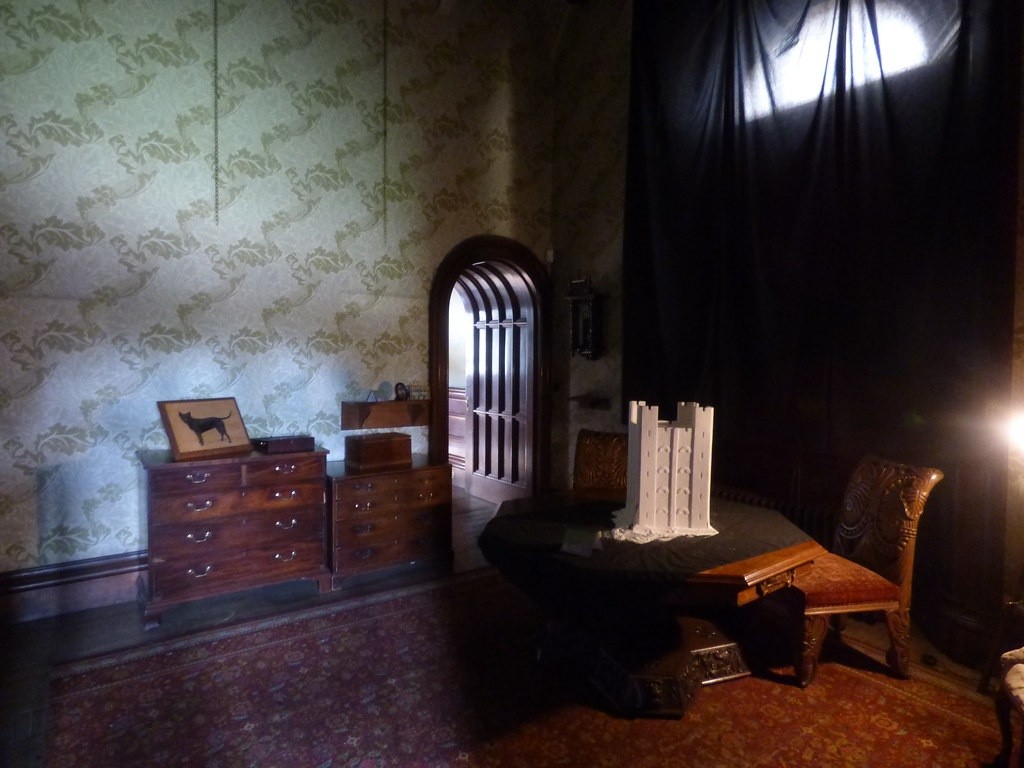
top-left (343, 431), bottom-right (414, 473)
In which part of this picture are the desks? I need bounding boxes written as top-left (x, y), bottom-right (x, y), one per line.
top-left (479, 490), bottom-right (828, 721)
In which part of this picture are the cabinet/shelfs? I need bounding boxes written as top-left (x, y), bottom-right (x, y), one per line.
top-left (129, 438), bottom-right (334, 633)
top-left (325, 453), bottom-right (456, 592)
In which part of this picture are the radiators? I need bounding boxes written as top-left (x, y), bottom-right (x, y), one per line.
top-left (712, 484), bottom-right (839, 551)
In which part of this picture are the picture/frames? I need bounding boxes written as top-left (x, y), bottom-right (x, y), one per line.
top-left (159, 396), bottom-right (255, 460)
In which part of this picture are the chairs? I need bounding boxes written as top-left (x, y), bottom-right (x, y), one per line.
top-left (756, 455), bottom-right (946, 688)
top-left (572, 428), bottom-right (629, 501)
